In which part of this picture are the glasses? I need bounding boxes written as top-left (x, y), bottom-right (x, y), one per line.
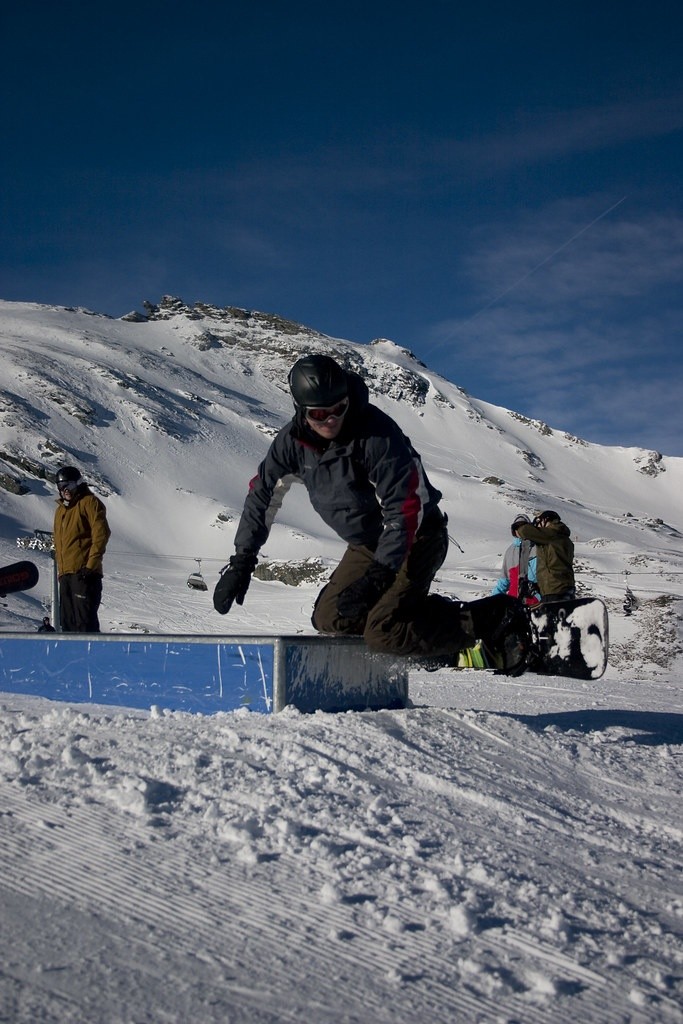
top-left (307, 397), bottom-right (349, 422)
top-left (58, 478), bottom-right (81, 490)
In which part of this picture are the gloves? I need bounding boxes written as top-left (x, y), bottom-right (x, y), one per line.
top-left (213, 553), bottom-right (260, 615)
top-left (75, 565), bottom-right (95, 582)
top-left (337, 560), bottom-right (398, 618)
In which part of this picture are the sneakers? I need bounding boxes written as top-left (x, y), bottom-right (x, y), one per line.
top-left (467, 594), bottom-right (534, 678)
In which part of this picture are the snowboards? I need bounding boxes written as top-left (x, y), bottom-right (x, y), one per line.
top-left (450, 594), bottom-right (611, 681)
top-left (0, 559), bottom-right (42, 597)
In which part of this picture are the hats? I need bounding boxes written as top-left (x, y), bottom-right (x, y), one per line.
top-left (511, 516), bottom-right (528, 527)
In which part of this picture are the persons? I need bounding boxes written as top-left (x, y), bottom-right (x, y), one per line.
top-left (492, 514), bottom-right (540, 598)
top-left (213, 355), bottom-right (531, 677)
top-left (50, 466), bottom-right (111, 632)
top-left (517, 510), bottom-right (576, 603)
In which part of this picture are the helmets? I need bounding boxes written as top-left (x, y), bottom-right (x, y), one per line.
top-left (537, 510), bottom-right (562, 523)
top-left (288, 355), bottom-right (349, 407)
top-left (56, 466), bottom-right (82, 483)
top-left (43, 617), bottom-right (50, 623)
